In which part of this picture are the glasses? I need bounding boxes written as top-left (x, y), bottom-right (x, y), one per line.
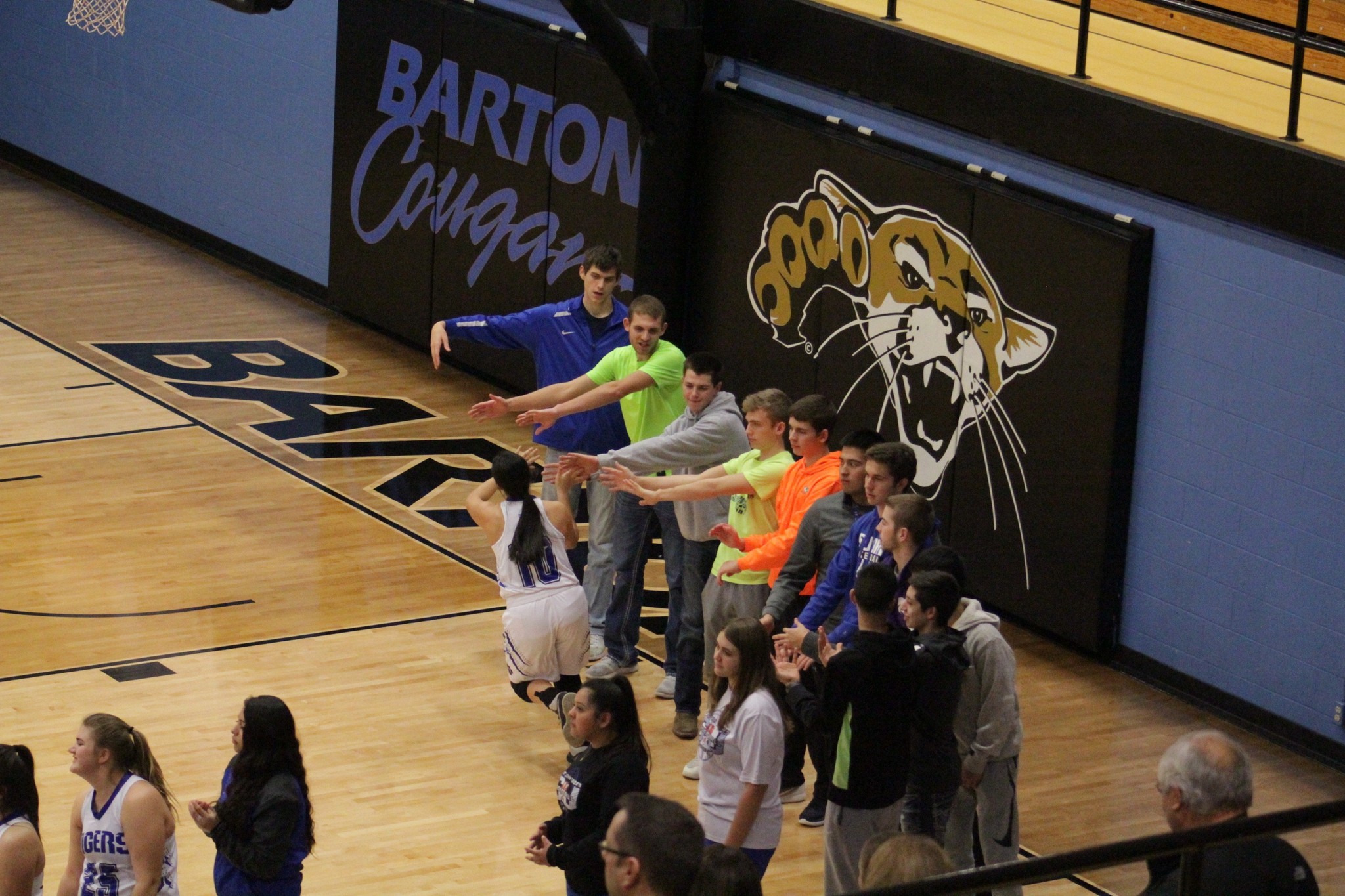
top-left (598, 840), bottom-right (630, 861)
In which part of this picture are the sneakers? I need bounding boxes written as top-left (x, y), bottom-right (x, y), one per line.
top-left (799, 798), bottom-right (828, 826)
top-left (779, 774), bottom-right (806, 803)
top-left (682, 756), bottom-right (700, 779)
top-left (673, 712), bottom-right (698, 737)
top-left (655, 676), bottom-right (675, 698)
top-left (589, 635), bottom-right (607, 660)
top-left (585, 657), bottom-right (638, 679)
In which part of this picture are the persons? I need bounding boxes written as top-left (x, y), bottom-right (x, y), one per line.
top-left (0, 743), bottom-right (46, 896)
top-left (429, 245), bottom-right (1021, 896)
top-left (466, 445), bottom-right (591, 764)
top-left (56, 712), bottom-right (181, 896)
top-left (598, 788), bottom-right (705, 896)
top-left (1137, 728), bottom-right (1320, 896)
top-left (697, 614), bottom-right (785, 881)
top-left (189, 695), bottom-right (316, 896)
top-left (523, 673), bottom-right (653, 896)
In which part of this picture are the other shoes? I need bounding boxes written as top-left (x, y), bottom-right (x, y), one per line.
top-left (557, 691), bottom-right (585, 748)
